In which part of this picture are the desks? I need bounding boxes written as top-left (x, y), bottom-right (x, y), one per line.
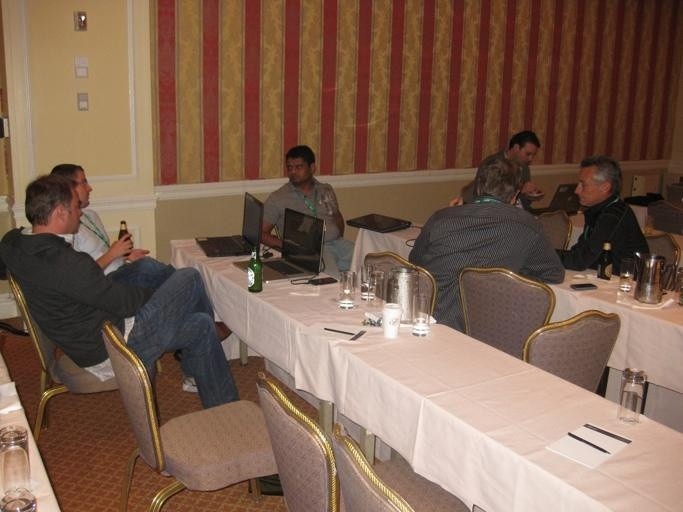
top-left (0, 351), bottom-right (62, 512)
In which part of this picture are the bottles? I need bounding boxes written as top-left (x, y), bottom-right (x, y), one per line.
top-left (248, 247), bottom-right (263, 292)
top-left (118, 220), bottom-right (132, 257)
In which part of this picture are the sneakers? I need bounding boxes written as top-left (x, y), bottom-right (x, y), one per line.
top-left (181, 375), bottom-right (200, 393)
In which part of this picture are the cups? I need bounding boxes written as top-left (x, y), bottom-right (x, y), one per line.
top-left (528, 183), bottom-right (543, 197)
top-left (0, 425), bottom-right (36, 512)
top-left (596, 240), bottom-right (683, 306)
top-left (615, 367), bottom-right (648, 424)
top-left (337, 262), bottom-right (432, 338)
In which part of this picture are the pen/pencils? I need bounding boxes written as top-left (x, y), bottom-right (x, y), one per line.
top-left (567, 432), bottom-right (611, 455)
top-left (324, 328), bottom-right (355, 335)
top-left (274, 224), bottom-right (281, 240)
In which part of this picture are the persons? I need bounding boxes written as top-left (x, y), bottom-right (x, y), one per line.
top-left (491, 132), bottom-right (541, 214)
top-left (552, 152), bottom-right (650, 281)
top-left (408, 156), bottom-right (564, 332)
top-left (51, 164), bottom-right (198, 393)
top-left (0, 175), bottom-right (239, 409)
top-left (261, 145), bottom-right (356, 281)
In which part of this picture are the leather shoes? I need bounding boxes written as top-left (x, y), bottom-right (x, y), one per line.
top-left (176, 319), bottom-right (232, 361)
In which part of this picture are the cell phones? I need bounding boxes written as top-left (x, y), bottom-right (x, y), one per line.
top-left (572, 283), bottom-right (596, 290)
top-left (312, 277), bottom-right (337, 285)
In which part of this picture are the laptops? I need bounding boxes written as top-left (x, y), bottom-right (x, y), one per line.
top-left (530, 183), bottom-right (581, 215)
top-left (196, 192), bottom-right (264, 258)
top-left (347, 214), bottom-right (411, 233)
top-left (233, 208), bottom-right (324, 284)
